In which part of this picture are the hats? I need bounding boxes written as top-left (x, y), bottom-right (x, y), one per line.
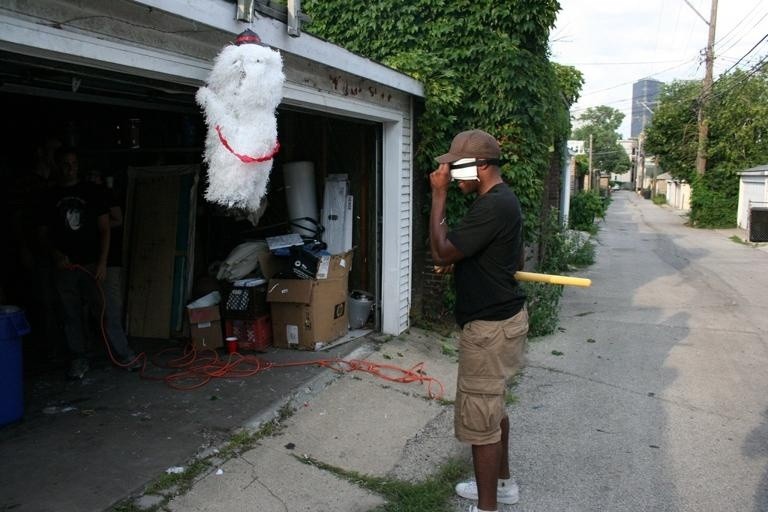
top-left (433, 130), bottom-right (500, 164)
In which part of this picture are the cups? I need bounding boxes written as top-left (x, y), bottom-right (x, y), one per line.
top-left (226, 337), bottom-right (238, 355)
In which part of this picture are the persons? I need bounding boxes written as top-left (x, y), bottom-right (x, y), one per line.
top-left (78, 157), bottom-right (146, 373)
top-left (426, 129), bottom-right (531, 512)
top-left (30, 150), bottom-right (114, 385)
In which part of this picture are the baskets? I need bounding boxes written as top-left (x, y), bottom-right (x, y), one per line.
top-left (220, 281), bottom-right (272, 352)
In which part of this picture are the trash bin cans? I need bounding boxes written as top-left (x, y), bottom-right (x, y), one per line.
top-left (0, 305), bottom-right (31, 429)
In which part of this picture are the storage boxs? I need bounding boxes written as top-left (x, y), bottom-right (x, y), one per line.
top-left (183, 307), bottom-right (223, 352)
top-left (266, 249), bottom-right (356, 351)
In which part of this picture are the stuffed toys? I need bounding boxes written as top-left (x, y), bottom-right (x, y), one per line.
top-left (193, 29), bottom-right (286, 213)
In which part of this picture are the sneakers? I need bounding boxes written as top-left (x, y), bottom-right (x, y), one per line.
top-left (68, 364), bottom-right (90, 379)
top-left (118, 356), bottom-right (142, 372)
top-left (455, 478), bottom-right (519, 504)
